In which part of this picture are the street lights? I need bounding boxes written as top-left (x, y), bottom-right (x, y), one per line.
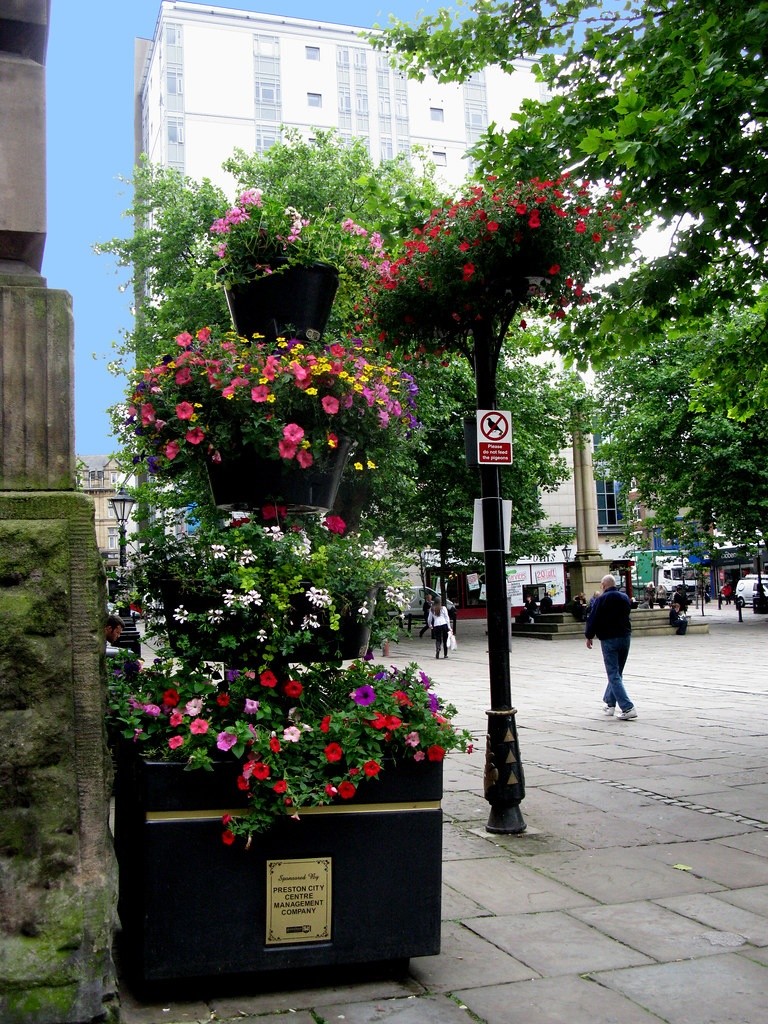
top-left (106, 485), bottom-right (141, 657)
top-left (562, 544), bottom-right (571, 604)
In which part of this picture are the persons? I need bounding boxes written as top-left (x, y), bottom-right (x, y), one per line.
top-left (519, 582), bottom-right (731, 636)
top-left (105, 615), bottom-right (125, 643)
top-left (584, 574), bottom-right (638, 719)
top-left (419, 594), bottom-right (436, 639)
top-left (428, 597), bottom-right (452, 659)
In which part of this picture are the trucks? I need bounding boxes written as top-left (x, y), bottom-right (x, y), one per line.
top-left (613, 550), bottom-right (699, 604)
top-left (735, 574), bottom-right (768, 607)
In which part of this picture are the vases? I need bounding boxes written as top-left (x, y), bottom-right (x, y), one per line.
top-left (216, 255), bottom-right (341, 356)
top-left (154, 571), bottom-right (269, 666)
top-left (274, 584), bottom-right (378, 660)
top-left (200, 437), bottom-right (359, 513)
top-left (111, 739), bottom-right (446, 1008)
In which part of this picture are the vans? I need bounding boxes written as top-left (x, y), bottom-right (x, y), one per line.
top-left (384, 586), bottom-right (455, 622)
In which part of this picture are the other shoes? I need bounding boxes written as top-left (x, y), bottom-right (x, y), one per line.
top-left (436, 649), bottom-right (439, 658)
top-left (444, 656), bottom-right (448, 658)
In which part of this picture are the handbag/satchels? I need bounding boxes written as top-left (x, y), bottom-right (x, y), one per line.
top-left (446, 631), bottom-right (457, 651)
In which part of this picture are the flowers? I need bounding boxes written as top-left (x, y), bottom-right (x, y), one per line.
top-left (95, 169), bottom-right (645, 848)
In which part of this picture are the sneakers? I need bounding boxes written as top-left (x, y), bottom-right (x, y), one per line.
top-left (616, 707), bottom-right (637, 719)
top-left (603, 706), bottom-right (615, 715)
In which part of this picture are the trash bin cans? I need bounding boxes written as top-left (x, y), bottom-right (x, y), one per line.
top-left (118, 620), bottom-right (142, 659)
top-left (753, 596), bottom-right (768, 613)
top-left (108, 580), bottom-right (120, 603)
